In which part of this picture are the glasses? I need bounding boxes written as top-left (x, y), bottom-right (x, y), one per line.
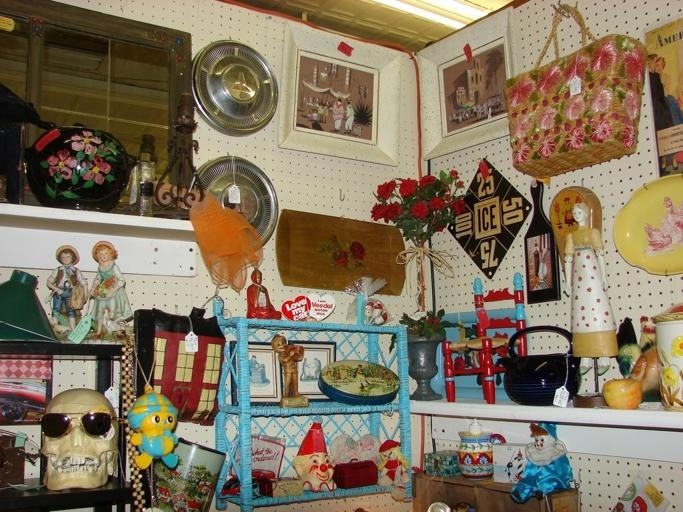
top-left (39, 411), bottom-right (115, 438)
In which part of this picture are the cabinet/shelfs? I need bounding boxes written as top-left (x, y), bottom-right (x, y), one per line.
top-left (212, 299), bottom-right (411, 512)
top-left (0, 339), bottom-right (132, 512)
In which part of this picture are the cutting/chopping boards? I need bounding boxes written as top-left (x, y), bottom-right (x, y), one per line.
top-left (276, 209), bottom-right (407, 296)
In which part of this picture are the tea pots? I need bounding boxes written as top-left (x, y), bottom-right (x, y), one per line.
top-left (456, 419), bottom-right (495, 480)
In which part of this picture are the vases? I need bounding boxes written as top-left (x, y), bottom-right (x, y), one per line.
top-left (408, 334), bottom-right (443, 400)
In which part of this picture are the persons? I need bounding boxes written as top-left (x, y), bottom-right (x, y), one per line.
top-left (647, 53), bottom-right (675, 177)
top-left (245, 267), bottom-right (280, 319)
top-left (123, 382), bottom-right (182, 475)
top-left (271, 333), bottom-right (305, 398)
top-left (89, 240), bottom-right (134, 341)
top-left (291, 416), bottom-right (338, 493)
top-left (655, 56), bottom-right (682, 171)
top-left (46, 241), bottom-right (87, 336)
top-left (375, 438), bottom-right (410, 502)
top-left (317, 96), bottom-right (356, 134)
top-left (508, 423), bottom-right (574, 503)
top-left (562, 199), bottom-right (620, 360)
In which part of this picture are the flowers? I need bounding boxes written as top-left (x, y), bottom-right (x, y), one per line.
top-left (389, 309), bottom-right (463, 354)
top-left (371, 171), bottom-right (467, 316)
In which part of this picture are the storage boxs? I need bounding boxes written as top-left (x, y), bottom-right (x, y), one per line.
top-left (413, 473), bottom-right (581, 512)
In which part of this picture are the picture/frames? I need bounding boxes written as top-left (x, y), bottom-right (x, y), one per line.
top-left (417, 5), bottom-right (520, 161)
top-left (278, 23), bottom-right (401, 166)
top-left (230, 341), bottom-right (283, 406)
top-left (287, 339), bottom-right (336, 401)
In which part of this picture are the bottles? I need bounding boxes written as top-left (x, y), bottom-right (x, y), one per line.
top-left (128, 152), bottom-right (155, 215)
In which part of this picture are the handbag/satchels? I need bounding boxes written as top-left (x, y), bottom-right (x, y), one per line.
top-left (503, 34), bottom-right (648, 178)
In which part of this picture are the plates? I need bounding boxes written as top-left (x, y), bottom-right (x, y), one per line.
top-left (613, 174), bottom-right (683, 275)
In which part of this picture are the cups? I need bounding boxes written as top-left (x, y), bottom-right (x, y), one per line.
top-left (652, 317), bottom-right (683, 411)
